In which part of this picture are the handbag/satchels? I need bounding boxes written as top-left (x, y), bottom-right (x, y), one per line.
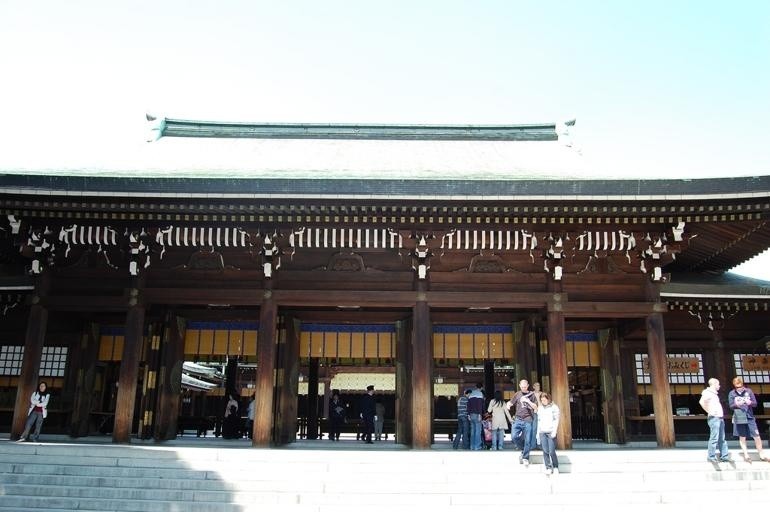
top-left (734, 408), bottom-right (748, 425)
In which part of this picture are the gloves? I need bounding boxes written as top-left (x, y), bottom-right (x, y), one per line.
top-left (360, 413), bottom-right (364, 419)
top-left (374, 415), bottom-right (378, 420)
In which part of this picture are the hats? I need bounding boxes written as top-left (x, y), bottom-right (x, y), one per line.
top-left (367, 385), bottom-right (374, 390)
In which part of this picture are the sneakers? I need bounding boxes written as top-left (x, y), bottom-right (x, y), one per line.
top-left (553, 469), bottom-right (558, 475)
top-left (523, 459), bottom-right (529, 466)
top-left (710, 457), bottom-right (769, 463)
top-left (361, 435), bottom-right (381, 444)
top-left (546, 469), bottom-right (551, 474)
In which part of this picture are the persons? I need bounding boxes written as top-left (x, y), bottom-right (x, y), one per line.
top-left (530, 381), bottom-right (544, 448)
top-left (487, 389), bottom-right (513, 450)
top-left (537, 391), bottom-right (561, 475)
top-left (246, 393), bottom-right (256, 440)
top-left (329, 387), bottom-right (347, 441)
top-left (224, 392), bottom-right (239, 438)
top-left (360, 384), bottom-right (376, 445)
top-left (452, 389), bottom-right (473, 449)
top-left (728, 377), bottom-right (769, 462)
top-left (360, 431), bottom-right (367, 440)
top-left (508, 378), bottom-right (536, 466)
top-left (698, 377), bottom-right (735, 463)
top-left (15, 380), bottom-right (52, 441)
top-left (374, 398), bottom-right (385, 441)
top-left (466, 383), bottom-right (483, 451)
